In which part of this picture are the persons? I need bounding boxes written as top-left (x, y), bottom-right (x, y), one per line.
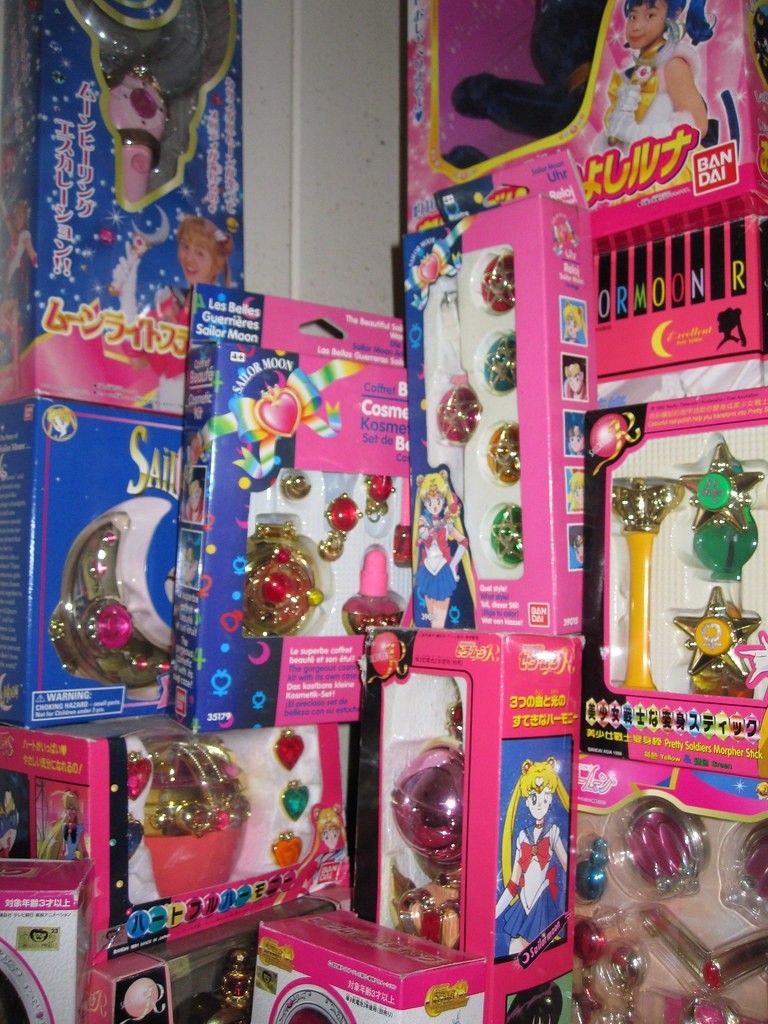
top-left (593, 0), bottom-right (713, 158)
top-left (113, 214), bottom-right (234, 413)
